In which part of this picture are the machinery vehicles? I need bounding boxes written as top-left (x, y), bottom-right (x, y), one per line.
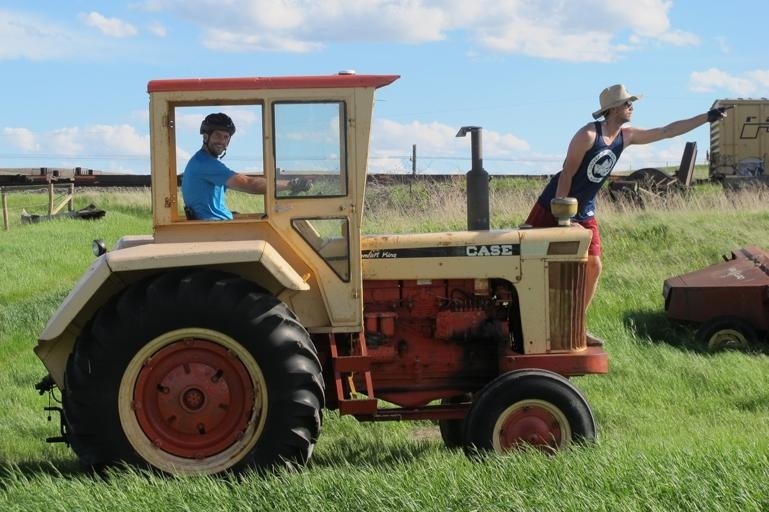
top-left (29, 62), bottom-right (615, 488)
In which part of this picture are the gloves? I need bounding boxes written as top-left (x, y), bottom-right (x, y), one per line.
top-left (708, 104), bottom-right (737, 123)
top-left (290, 177), bottom-right (313, 192)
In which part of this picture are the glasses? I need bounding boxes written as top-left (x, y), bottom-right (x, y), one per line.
top-left (624, 101), bottom-right (632, 109)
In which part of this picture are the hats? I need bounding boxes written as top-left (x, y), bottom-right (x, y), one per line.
top-left (592, 84), bottom-right (644, 120)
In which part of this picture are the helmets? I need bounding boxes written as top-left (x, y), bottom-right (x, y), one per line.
top-left (200, 113), bottom-right (235, 136)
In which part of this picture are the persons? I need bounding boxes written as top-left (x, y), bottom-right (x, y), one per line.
top-left (182, 113), bottom-right (313, 222)
top-left (523, 85), bottom-right (736, 347)
